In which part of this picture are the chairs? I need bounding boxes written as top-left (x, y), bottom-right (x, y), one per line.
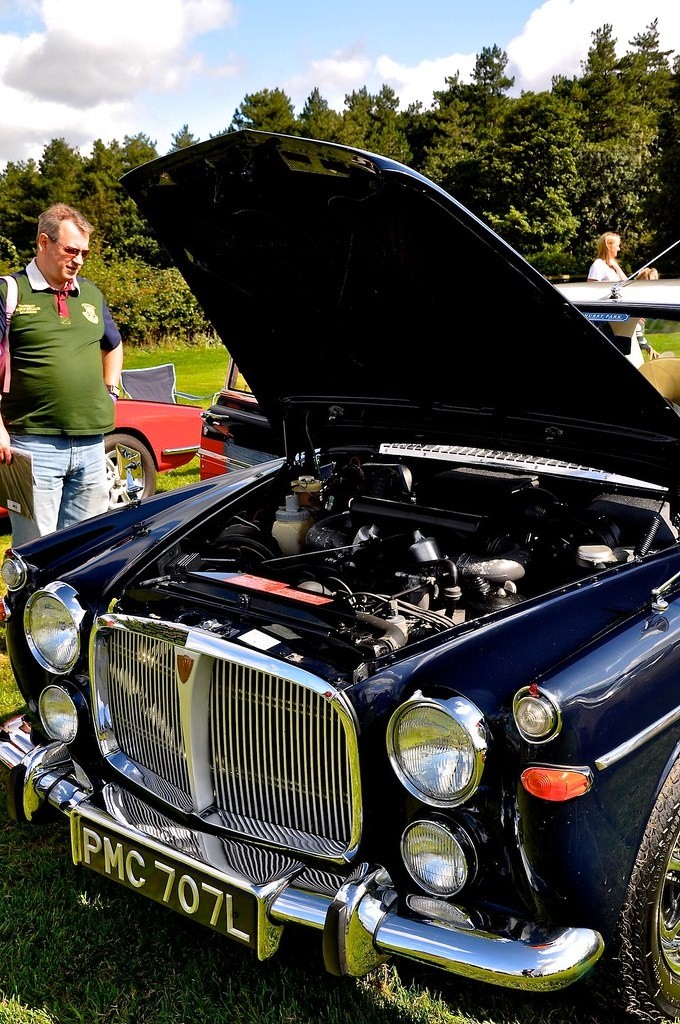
top-left (639, 357), bottom-right (680, 407)
top-left (118, 363), bottom-right (220, 406)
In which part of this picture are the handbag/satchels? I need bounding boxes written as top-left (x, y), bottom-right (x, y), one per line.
top-left (0, 275), bottom-right (18, 394)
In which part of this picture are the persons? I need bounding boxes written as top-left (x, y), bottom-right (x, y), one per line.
top-left (0, 203), bottom-right (124, 549)
top-left (586, 232), bottom-right (629, 282)
top-left (632, 266), bottom-right (661, 360)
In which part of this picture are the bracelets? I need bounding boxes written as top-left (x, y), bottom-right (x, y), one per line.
top-left (106, 385), bottom-right (121, 398)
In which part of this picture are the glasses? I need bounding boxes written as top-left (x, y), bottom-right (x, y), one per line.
top-left (46, 232), bottom-right (90, 258)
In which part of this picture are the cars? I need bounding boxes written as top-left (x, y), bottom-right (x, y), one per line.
top-left (0, 128), bottom-right (679, 1024)
